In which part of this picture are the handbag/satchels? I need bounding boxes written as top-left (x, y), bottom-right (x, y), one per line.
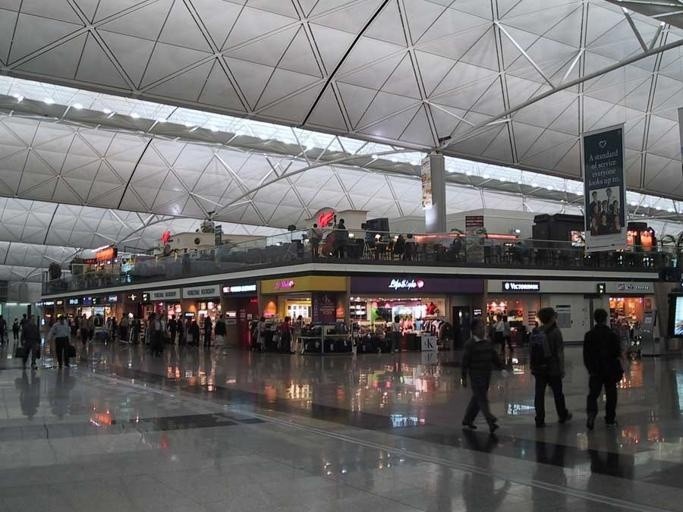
top-left (69, 345), bottom-right (76, 357)
top-left (15, 348), bottom-right (24, 357)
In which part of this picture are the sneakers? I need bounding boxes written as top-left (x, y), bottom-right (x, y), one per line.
top-left (31, 364), bottom-right (37, 369)
top-left (559, 413), bottom-right (572, 423)
top-left (586, 411), bottom-right (595, 428)
top-left (490, 424), bottom-right (498, 432)
top-left (607, 421), bottom-right (616, 427)
top-left (463, 421), bottom-right (477, 429)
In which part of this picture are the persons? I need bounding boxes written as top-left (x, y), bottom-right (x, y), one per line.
top-left (482, 233), bottom-right (492, 265)
top-left (586, 426), bottom-right (634, 511)
top-left (492, 314), bottom-right (504, 355)
top-left (530, 429), bottom-right (567, 512)
top-left (333, 218), bottom-right (347, 258)
top-left (589, 188), bottom-right (620, 235)
top-left (458, 429), bottom-right (511, 511)
top-left (443, 232), bottom-right (464, 257)
top-left (47, 368), bottom-right (70, 420)
top-left (278, 317), bottom-right (294, 355)
top-left (0, 313), bottom-right (228, 374)
top-left (501, 316), bottom-right (513, 353)
top-left (460, 316), bottom-right (502, 432)
top-left (514, 241), bottom-right (522, 248)
top-left (254, 317), bottom-right (266, 353)
top-left (528, 306), bottom-right (572, 429)
top-left (14, 370), bottom-right (40, 420)
top-left (581, 308), bottom-right (624, 431)
top-left (308, 223), bottom-right (321, 256)
top-left (296, 315), bottom-right (304, 329)
top-left (389, 314), bottom-right (404, 355)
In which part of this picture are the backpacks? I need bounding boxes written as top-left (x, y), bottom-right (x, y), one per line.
top-left (530, 324), bottom-right (558, 364)
